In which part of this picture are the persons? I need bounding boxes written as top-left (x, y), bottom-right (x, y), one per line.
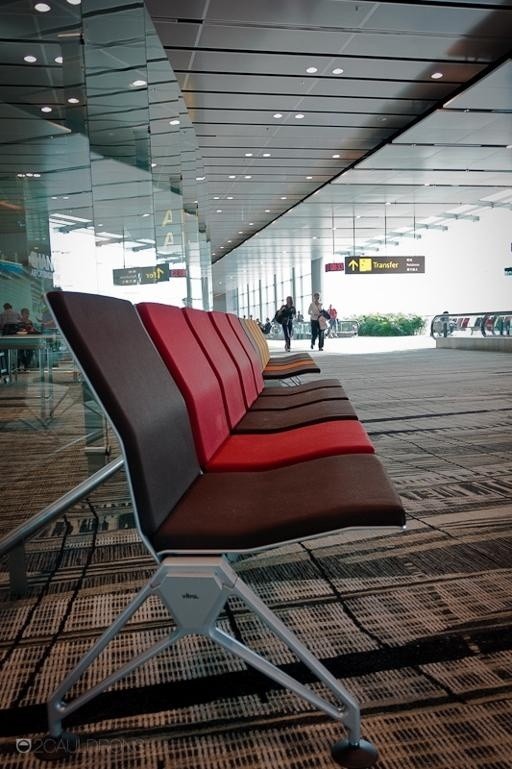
top-left (0, 296), bottom-right (56, 371)
top-left (244, 293), bottom-right (337, 352)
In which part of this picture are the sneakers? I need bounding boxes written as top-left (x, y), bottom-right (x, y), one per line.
top-left (311, 344), bottom-right (323, 351)
top-left (285, 343), bottom-right (291, 352)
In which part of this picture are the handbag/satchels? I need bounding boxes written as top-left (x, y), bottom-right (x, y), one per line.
top-left (319, 315), bottom-right (330, 331)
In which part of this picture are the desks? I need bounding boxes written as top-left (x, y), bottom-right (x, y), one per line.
top-left (0, 334), bottom-right (56, 384)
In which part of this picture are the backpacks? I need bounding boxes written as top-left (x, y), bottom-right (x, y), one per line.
top-left (275, 305), bottom-right (288, 324)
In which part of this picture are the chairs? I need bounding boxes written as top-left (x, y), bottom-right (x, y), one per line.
top-left (30, 290), bottom-right (406, 765)
top-left (446, 316), bottom-right (511, 336)
